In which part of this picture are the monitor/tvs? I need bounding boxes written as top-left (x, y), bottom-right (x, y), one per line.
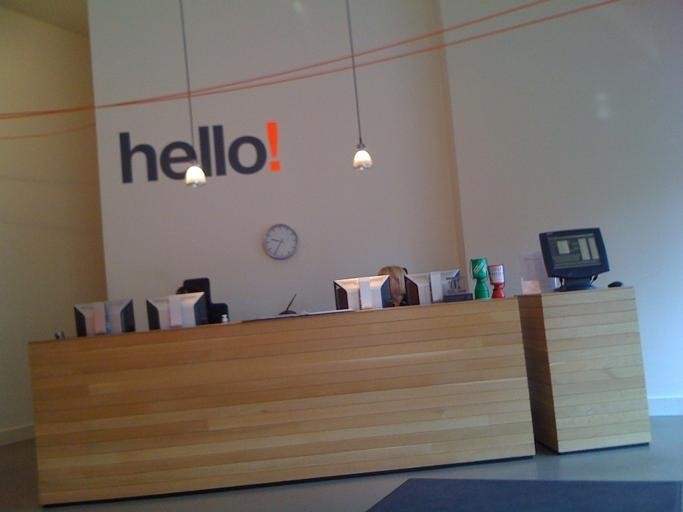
top-left (539, 227), bottom-right (610, 292)
top-left (404, 269), bottom-right (463, 305)
top-left (146, 291), bottom-right (209, 330)
top-left (333, 275), bottom-right (394, 310)
top-left (73, 297), bottom-right (135, 337)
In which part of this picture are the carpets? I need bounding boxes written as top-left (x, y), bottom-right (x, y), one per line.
top-left (364, 478), bottom-right (683, 512)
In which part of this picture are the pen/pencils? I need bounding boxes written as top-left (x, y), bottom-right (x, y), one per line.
top-left (286, 294), bottom-right (296, 311)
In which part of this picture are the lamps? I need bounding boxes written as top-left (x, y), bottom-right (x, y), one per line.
top-left (343, 0), bottom-right (374, 171)
top-left (178, 1), bottom-right (207, 190)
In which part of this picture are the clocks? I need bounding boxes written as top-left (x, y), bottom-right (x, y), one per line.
top-left (260, 224), bottom-right (298, 261)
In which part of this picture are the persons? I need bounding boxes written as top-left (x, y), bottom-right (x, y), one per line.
top-left (378, 266), bottom-right (407, 308)
